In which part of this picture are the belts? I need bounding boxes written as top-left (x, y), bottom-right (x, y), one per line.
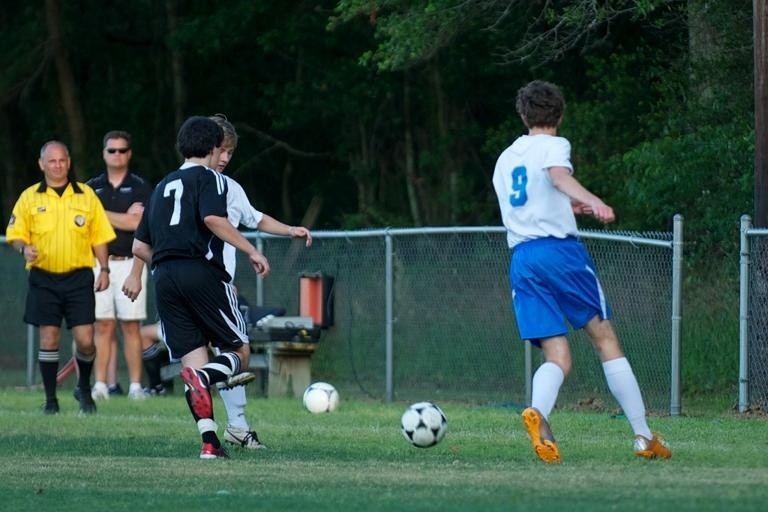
top-left (104, 253), bottom-right (133, 262)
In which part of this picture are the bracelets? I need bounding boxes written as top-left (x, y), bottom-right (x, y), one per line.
top-left (101, 267), bottom-right (110, 273)
top-left (18, 244), bottom-right (25, 256)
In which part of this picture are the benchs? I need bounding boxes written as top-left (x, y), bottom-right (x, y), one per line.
top-left (159, 339), bottom-right (316, 400)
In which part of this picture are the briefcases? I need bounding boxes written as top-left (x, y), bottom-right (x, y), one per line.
top-left (247, 269), bottom-right (335, 342)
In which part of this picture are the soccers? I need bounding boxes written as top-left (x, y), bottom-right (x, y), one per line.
top-left (401, 401), bottom-right (448, 448)
top-left (303, 381), bottom-right (339, 413)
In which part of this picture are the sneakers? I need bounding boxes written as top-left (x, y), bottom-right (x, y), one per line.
top-left (521, 407), bottom-right (560, 463)
top-left (216, 371), bottom-right (255, 391)
top-left (46, 383), bottom-right (166, 414)
top-left (180, 366), bottom-right (213, 418)
top-left (632, 431), bottom-right (672, 459)
top-left (199, 441), bottom-right (230, 459)
top-left (223, 428), bottom-right (267, 449)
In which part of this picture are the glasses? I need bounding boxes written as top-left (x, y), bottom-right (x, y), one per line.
top-left (107, 147), bottom-right (130, 153)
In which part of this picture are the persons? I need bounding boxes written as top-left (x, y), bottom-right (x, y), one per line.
top-left (121, 114), bottom-right (313, 450)
top-left (5, 140), bottom-right (117, 415)
top-left (137, 314), bottom-right (171, 400)
top-left (487, 79), bottom-right (675, 460)
top-left (68, 330), bottom-right (124, 397)
top-left (82, 131), bottom-right (152, 403)
top-left (130, 114), bottom-right (274, 461)
